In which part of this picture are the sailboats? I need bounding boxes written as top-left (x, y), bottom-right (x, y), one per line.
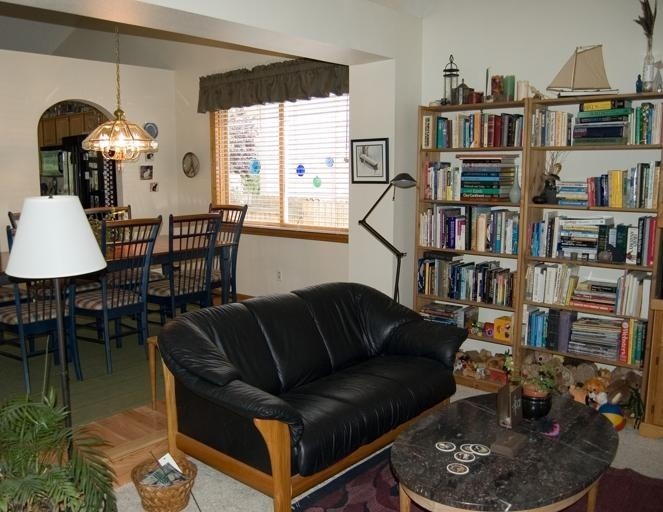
top-left (546, 45), bottom-right (621, 98)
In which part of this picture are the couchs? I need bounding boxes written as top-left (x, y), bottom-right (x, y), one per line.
top-left (158, 279), bottom-right (472, 512)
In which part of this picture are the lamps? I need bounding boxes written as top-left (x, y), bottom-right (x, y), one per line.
top-left (356, 172), bottom-right (419, 300)
top-left (81, 25), bottom-right (159, 163)
top-left (2, 193), bottom-right (111, 464)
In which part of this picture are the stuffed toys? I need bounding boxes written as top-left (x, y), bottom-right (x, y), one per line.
top-left (451, 346), bottom-right (514, 386)
top-left (519, 348), bottom-right (647, 411)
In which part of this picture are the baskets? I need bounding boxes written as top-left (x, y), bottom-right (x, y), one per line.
top-left (131, 455), bottom-right (198, 512)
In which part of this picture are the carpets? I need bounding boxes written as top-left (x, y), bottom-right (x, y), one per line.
top-left (288, 441), bottom-right (663, 510)
top-left (97, 385), bottom-right (663, 511)
top-left (0, 285), bottom-right (201, 445)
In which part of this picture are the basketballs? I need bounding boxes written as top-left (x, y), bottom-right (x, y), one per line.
top-left (598, 402), bottom-right (626, 431)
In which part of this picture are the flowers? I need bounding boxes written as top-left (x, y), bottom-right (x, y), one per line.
top-left (502, 350), bottom-right (561, 387)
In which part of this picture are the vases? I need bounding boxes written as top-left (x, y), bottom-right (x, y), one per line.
top-left (508, 165), bottom-right (522, 204)
top-left (640, 36), bottom-right (656, 91)
top-left (521, 390), bottom-right (554, 421)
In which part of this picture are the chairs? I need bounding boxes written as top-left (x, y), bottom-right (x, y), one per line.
top-left (66, 215), bottom-right (163, 373)
top-left (181, 202), bottom-right (247, 303)
top-left (9, 274), bottom-right (83, 396)
top-left (136, 211), bottom-right (221, 326)
top-left (1, 205), bottom-right (164, 304)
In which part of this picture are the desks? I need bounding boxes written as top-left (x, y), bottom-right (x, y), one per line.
top-left (1, 236), bottom-right (237, 364)
top-left (386, 391), bottom-right (620, 512)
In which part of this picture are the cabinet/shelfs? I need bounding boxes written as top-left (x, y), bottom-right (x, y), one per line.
top-left (414, 98), bottom-right (528, 395)
top-left (65, 107), bottom-right (102, 136)
top-left (512, 92), bottom-right (662, 439)
top-left (36, 111), bottom-right (72, 149)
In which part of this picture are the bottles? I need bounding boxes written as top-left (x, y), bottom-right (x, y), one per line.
top-left (507, 165), bottom-right (521, 204)
top-left (451, 73), bottom-right (514, 105)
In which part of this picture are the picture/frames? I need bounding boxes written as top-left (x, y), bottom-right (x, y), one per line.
top-left (350, 136), bottom-right (390, 184)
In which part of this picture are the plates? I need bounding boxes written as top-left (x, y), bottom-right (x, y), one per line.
top-left (182, 152), bottom-right (200, 178)
top-left (144, 124), bottom-right (158, 140)
